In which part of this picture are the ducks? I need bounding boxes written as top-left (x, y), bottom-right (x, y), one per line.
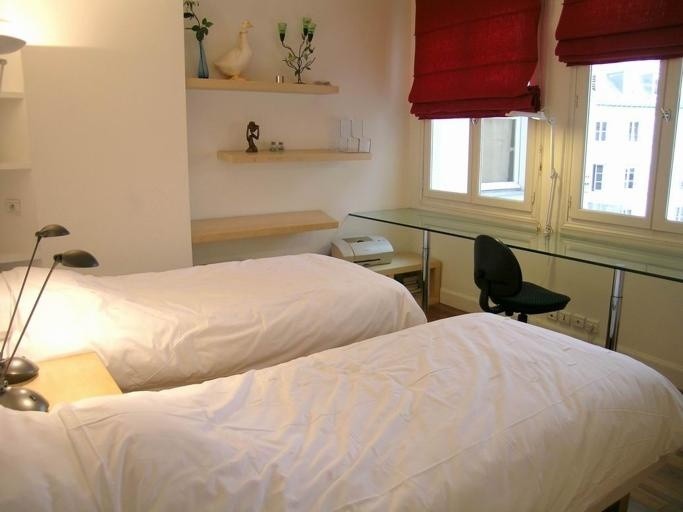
top-left (213, 18), bottom-right (255, 80)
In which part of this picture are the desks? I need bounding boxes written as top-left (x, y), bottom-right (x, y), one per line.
top-left (348, 207), bottom-right (683, 353)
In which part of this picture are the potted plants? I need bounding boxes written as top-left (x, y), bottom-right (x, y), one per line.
top-left (182, 0), bottom-right (214, 78)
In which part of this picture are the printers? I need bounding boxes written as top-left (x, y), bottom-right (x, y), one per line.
top-left (331, 235), bottom-right (393, 267)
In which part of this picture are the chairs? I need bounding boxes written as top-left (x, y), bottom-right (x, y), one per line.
top-left (473, 234), bottom-right (571, 327)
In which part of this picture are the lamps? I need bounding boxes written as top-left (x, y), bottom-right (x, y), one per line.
top-left (0, 221), bottom-right (97, 412)
